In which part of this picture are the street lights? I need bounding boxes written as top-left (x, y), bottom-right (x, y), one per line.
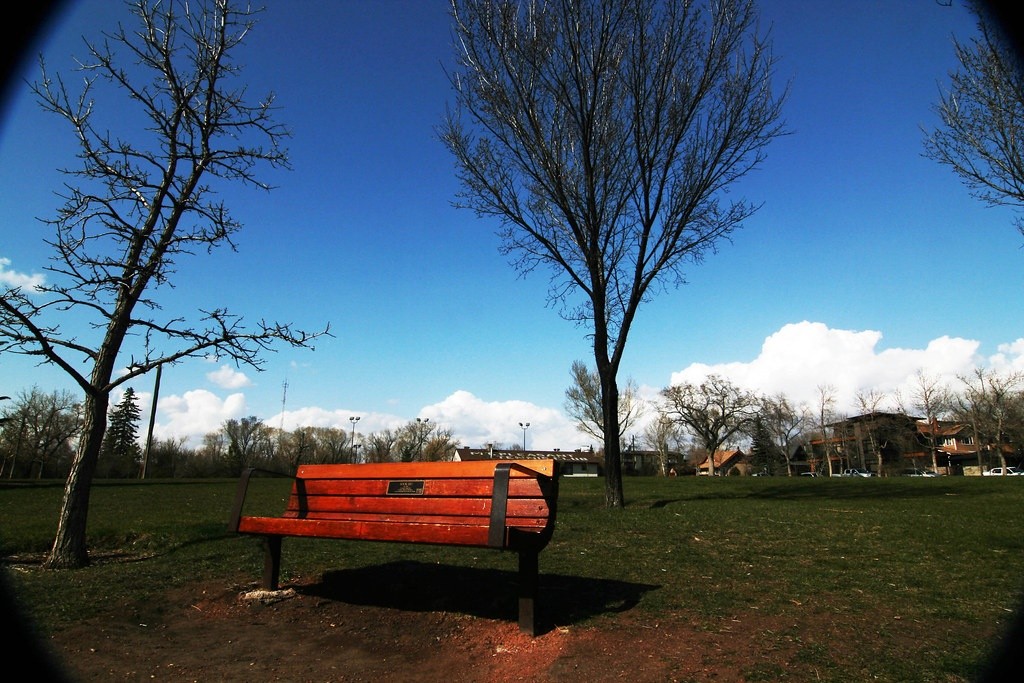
top-left (349, 417), bottom-right (361, 463)
top-left (520, 422), bottom-right (530, 450)
top-left (417, 417), bottom-right (429, 460)
top-left (353, 445), bottom-right (361, 462)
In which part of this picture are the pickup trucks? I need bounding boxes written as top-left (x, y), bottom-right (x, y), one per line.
top-left (832, 468), bottom-right (877, 477)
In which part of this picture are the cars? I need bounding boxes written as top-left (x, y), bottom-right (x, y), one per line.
top-left (894, 467), bottom-right (940, 477)
top-left (983, 467), bottom-right (1023, 475)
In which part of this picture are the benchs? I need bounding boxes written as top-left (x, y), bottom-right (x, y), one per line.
top-left (227, 459), bottom-right (559, 635)
